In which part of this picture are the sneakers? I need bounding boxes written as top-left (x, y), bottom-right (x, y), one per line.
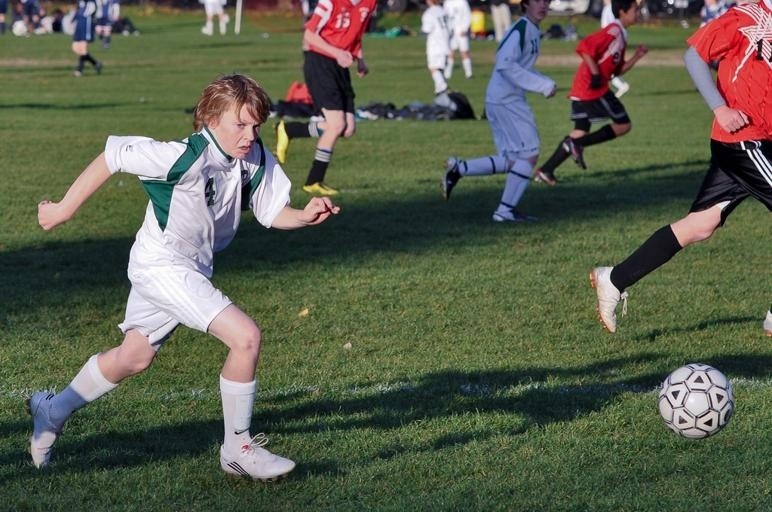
top-left (493, 209), bottom-right (538, 223)
top-left (533, 168), bottom-right (558, 186)
top-left (220, 439), bottom-right (295, 483)
top-left (433, 83), bottom-right (450, 95)
top-left (75, 61), bottom-right (106, 77)
top-left (588, 264), bottom-right (630, 335)
top-left (272, 120), bottom-right (290, 165)
top-left (302, 181), bottom-right (338, 196)
top-left (562, 135), bottom-right (588, 170)
top-left (442, 156), bottom-right (464, 202)
top-left (26, 389), bottom-right (65, 469)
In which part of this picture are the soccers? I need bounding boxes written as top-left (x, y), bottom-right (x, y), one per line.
top-left (658, 363), bottom-right (737, 437)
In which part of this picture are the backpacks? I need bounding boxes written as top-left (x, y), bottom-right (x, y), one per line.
top-left (286, 80), bottom-right (313, 104)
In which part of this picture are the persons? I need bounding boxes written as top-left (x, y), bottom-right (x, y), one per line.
top-left (417, 1), bottom-right (514, 95)
top-left (588, 0), bottom-right (772, 335)
top-left (0, 0), bottom-right (140, 76)
top-left (443, 0), bottom-right (556, 224)
top-left (535, 0), bottom-right (649, 186)
top-left (276, 0), bottom-right (376, 195)
top-left (600, 0), bottom-right (732, 99)
top-left (21, 74), bottom-right (341, 481)
top-left (199, 0), bottom-right (227, 36)
